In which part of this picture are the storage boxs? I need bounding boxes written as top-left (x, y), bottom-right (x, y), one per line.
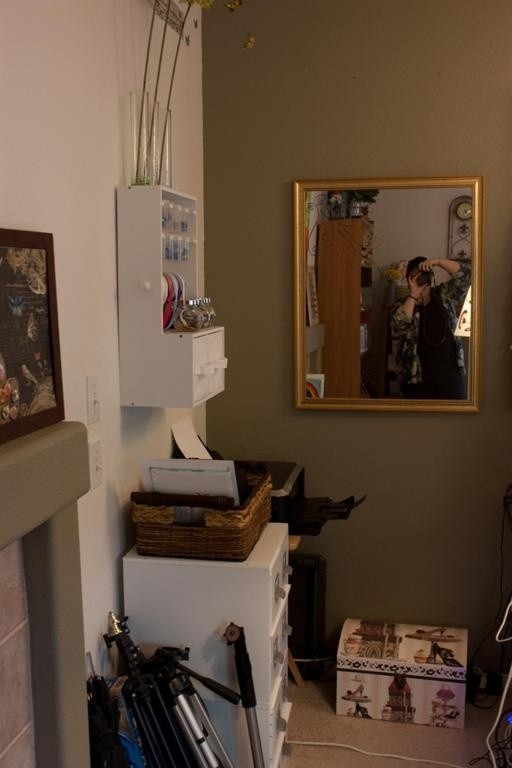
top-left (334, 620), bottom-right (471, 730)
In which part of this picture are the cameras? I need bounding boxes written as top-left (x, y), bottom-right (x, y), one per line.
top-left (416, 269), bottom-right (434, 287)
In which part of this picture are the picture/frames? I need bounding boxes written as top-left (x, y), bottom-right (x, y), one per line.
top-left (1, 226), bottom-right (65, 443)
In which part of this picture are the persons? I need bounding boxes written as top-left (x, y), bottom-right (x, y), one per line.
top-left (389, 254), bottom-right (472, 400)
top-left (407, 293), bottom-right (419, 301)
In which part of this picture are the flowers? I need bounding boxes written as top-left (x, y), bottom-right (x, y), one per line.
top-left (380, 267), bottom-right (402, 282)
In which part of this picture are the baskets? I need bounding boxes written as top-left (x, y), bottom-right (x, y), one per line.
top-left (131, 472), bottom-right (273, 560)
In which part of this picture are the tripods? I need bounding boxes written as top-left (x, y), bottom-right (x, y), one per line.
top-left (100, 610), bottom-right (232, 767)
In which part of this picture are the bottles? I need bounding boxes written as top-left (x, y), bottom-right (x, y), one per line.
top-left (173, 297), bottom-right (216, 333)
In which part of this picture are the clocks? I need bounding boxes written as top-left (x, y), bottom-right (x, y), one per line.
top-left (454, 200), bottom-right (475, 221)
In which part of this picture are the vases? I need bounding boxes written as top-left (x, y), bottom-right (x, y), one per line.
top-left (381, 281), bottom-right (396, 307)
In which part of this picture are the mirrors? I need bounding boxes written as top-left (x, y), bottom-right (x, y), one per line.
top-left (293, 177), bottom-right (484, 412)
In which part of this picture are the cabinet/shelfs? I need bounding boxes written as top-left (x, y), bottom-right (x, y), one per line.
top-left (115, 187), bottom-right (227, 410)
top-left (118, 523), bottom-right (298, 768)
top-left (316, 215), bottom-right (389, 397)
